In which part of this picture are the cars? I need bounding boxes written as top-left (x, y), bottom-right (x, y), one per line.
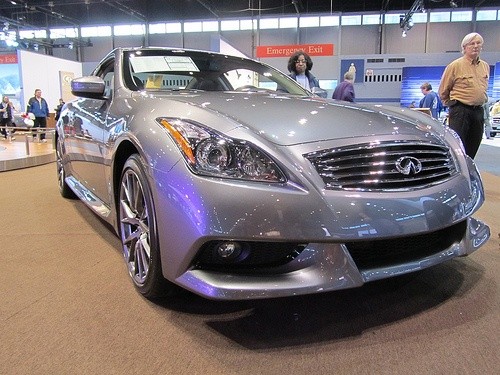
top-left (0, 108), bottom-right (28, 135)
top-left (52, 46), bottom-right (491, 302)
top-left (485, 99), bottom-right (500, 138)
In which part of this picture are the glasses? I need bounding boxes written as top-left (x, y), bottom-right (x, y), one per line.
top-left (295, 60), bottom-right (307, 63)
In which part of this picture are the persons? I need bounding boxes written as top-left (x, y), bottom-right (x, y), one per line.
top-left (54, 97), bottom-right (65, 121)
top-left (287, 51), bottom-right (320, 92)
top-left (419, 82), bottom-right (442, 120)
top-left (0, 96), bottom-right (17, 141)
top-left (27, 88), bottom-right (50, 143)
top-left (438, 32), bottom-right (489, 161)
top-left (332, 71), bottom-right (356, 102)
top-left (483, 99), bottom-right (500, 139)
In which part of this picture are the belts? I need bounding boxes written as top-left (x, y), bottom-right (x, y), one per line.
top-left (456, 101), bottom-right (482, 110)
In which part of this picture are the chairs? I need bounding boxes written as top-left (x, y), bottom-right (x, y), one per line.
top-left (133, 76), bottom-right (144, 88)
top-left (185, 76), bottom-right (229, 91)
